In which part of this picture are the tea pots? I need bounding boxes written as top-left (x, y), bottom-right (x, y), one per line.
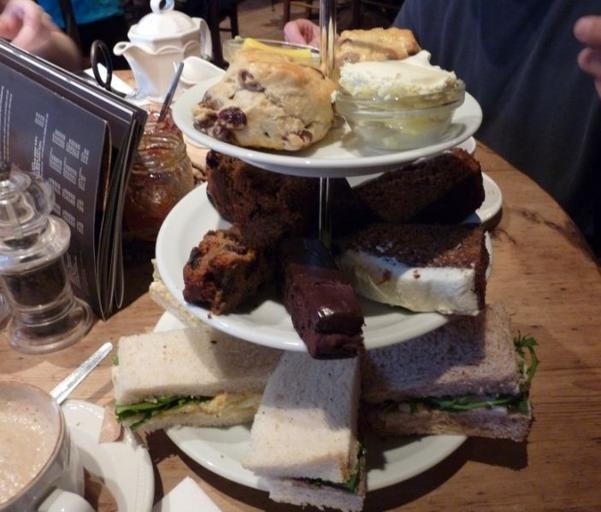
top-left (109, 1), bottom-right (216, 98)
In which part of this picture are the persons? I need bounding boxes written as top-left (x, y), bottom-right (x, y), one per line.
top-left (283, 1), bottom-right (601, 264)
top-left (0, 1), bottom-right (236, 71)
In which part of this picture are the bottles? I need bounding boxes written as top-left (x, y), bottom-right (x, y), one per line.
top-left (1, 170), bottom-right (92, 350)
top-left (122, 101), bottom-right (195, 243)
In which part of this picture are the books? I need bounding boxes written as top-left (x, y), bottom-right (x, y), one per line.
top-left (0, 36), bottom-right (147, 320)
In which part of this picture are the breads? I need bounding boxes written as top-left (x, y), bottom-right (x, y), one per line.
top-left (241, 352), bottom-right (362, 509)
top-left (149, 259), bottom-right (211, 328)
top-left (334, 28), bottom-right (421, 78)
top-left (278, 238), bottom-right (366, 359)
top-left (193, 48), bottom-right (336, 151)
top-left (332, 223), bottom-right (491, 316)
top-left (358, 305), bottom-right (540, 443)
top-left (339, 49), bottom-right (465, 147)
top-left (331, 147), bottom-right (486, 234)
top-left (183, 218), bottom-right (299, 316)
top-left (110, 329), bottom-right (283, 445)
top-left (241, 37), bottom-right (312, 61)
top-left (206, 151), bottom-right (364, 239)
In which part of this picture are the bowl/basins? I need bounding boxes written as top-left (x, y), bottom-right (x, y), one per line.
top-left (337, 84), bottom-right (470, 151)
top-left (1, 375), bottom-right (84, 512)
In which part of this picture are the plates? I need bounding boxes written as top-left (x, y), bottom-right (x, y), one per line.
top-left (49, 397), bottom-right (158, 512)
